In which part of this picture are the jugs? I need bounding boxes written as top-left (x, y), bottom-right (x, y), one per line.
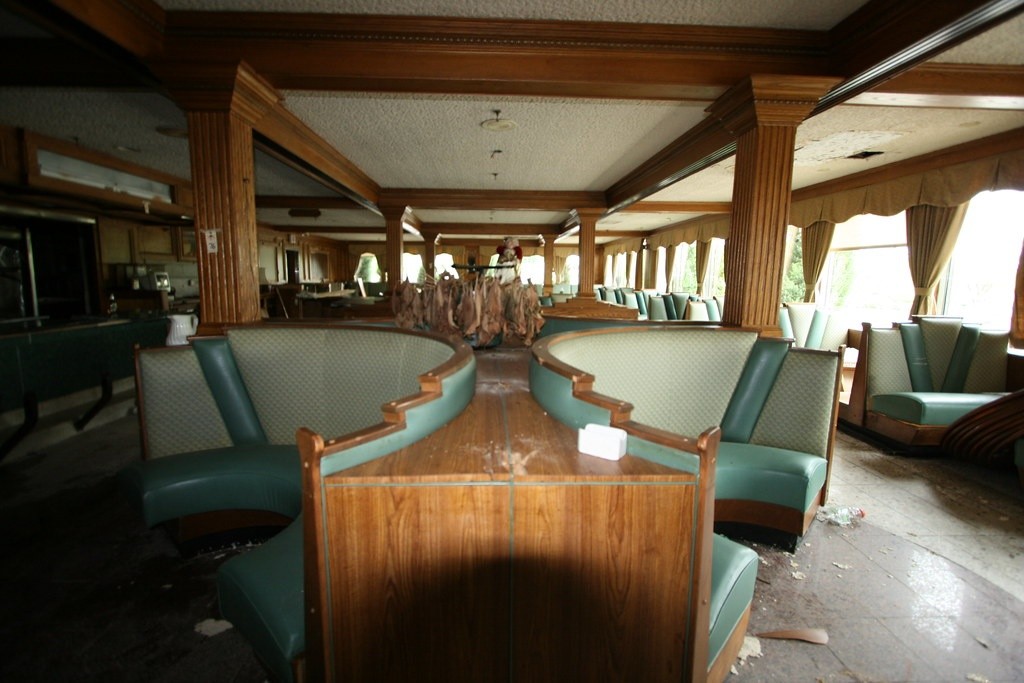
top-left (166, 313), bottom-right (198, 345)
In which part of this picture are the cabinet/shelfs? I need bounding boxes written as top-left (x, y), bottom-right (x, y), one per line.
top-left (174, 224), bottom-right (196, 262)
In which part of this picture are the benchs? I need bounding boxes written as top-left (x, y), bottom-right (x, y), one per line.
top-left (132, 318), bottom-right (478, 683)
top-left (838, 315), bottom-right (1015, 456)
top-left (523, 282), bottom-right (860, 369)
top-left (276, 280), bottom-right (388, 321)
top-left (536, 320), bottom-right (845, 683)
